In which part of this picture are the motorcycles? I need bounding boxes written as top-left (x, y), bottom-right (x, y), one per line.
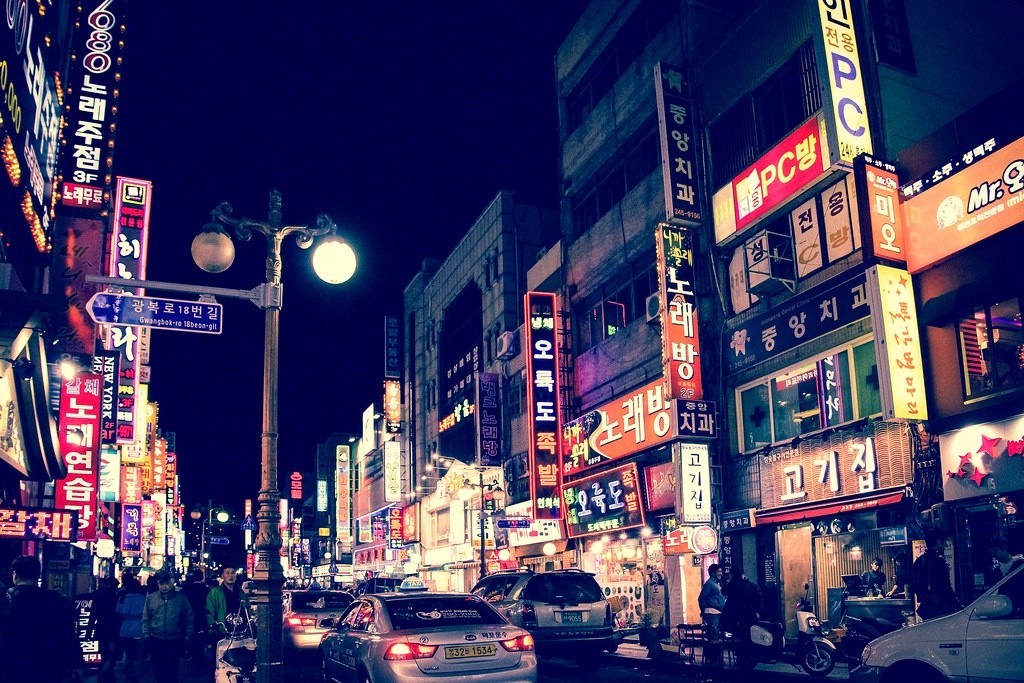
top-left (840, 605), bottom-right (917, 662)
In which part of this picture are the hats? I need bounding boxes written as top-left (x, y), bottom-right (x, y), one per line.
top-left (156, 573), bottom-right (171, 580)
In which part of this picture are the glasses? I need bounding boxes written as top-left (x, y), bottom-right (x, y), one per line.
top-left (158, 580), bottom-right (169, 585)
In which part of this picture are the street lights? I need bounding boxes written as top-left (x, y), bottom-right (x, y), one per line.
top-left (460, 468), bottom-right (504, 579)
top-left (191, 503), bottom-right (229, 575)
top-left (189, 186), bottom-right (358, 683)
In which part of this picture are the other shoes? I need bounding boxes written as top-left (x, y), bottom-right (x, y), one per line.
top-left (711, 659), bottom-right (725, 665)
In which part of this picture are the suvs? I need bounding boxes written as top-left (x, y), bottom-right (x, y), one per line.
top-left (469, 568), bottom-right (613, 663)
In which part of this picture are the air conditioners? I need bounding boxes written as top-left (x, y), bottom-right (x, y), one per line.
top-left (497, 331), bottom-right (514, 359)
top-left (646, 291), bottom-right (661, 324)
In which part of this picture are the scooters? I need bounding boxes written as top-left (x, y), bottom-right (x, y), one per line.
top-left (728, 583), bottom-right (837, 677)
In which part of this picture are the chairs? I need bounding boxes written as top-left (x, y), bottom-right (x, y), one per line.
top-left (676, 624), bottom-right (738, 667)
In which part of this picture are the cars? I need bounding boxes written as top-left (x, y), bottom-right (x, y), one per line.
top-left (280, 590), bottom-right (356, 651)
top-left (241, 581), bottom-right (257, 603)
top-left (849, 564), bottom-right (1024, 683)
top-left (314, 576), bottom-right (537, 683)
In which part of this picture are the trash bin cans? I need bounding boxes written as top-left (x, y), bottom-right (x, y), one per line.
top-left (843, 597), bottom-right (912, 675)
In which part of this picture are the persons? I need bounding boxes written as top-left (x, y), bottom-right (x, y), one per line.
top-left (0, 529), bottom-right (258, 683)
top-left (855, 526), bottom-right (1023, 621)
top-left (697, 562), bottom-right (768, 683)
